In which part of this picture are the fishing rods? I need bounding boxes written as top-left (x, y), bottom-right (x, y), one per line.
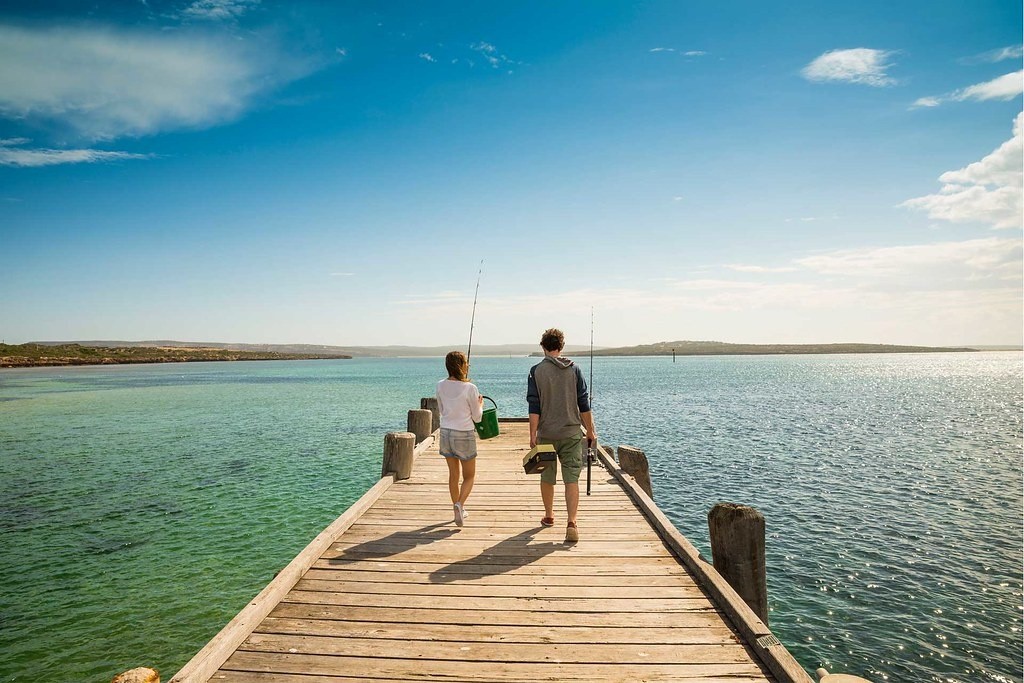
top-left (465, 260), bottom-right (484, 382)
top-left (586, 305), bottom-right (595, 496)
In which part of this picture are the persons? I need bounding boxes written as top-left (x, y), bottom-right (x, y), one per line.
top-left (527, 328), bottom-right (597, 542)
top-left (435, 351), bottom-right (483, 528)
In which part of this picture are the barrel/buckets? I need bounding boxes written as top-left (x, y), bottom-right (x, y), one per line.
top-left (472, 396), bottom-right (499, 440)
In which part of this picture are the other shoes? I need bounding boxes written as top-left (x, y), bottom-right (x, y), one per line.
top-left (566, 522), bottom-right (578, 541)
top-left (542, 517), bottom-right (553, 525)
top-left (463, 511), bottom-right (468, 518)
top-left (454, 503), bottom-right (463, 527)
